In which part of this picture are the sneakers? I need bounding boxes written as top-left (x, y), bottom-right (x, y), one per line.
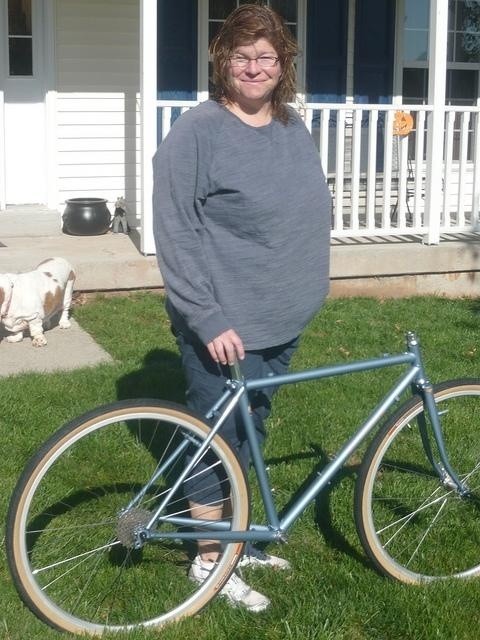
top-left (189, 541), bottom-right (290, 613)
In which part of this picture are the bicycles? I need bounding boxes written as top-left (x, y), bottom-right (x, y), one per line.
top-left (6, 331), bottom-right (480, 640)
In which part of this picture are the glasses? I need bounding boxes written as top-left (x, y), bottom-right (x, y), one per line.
top-left (226, 52), bottom-right (280, 67)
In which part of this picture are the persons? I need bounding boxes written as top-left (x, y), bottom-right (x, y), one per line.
top-left (150, 3), bottom-right (334, 615)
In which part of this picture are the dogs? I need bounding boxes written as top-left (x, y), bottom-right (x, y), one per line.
top-left (0, 255), bottom-right (77, 347)
